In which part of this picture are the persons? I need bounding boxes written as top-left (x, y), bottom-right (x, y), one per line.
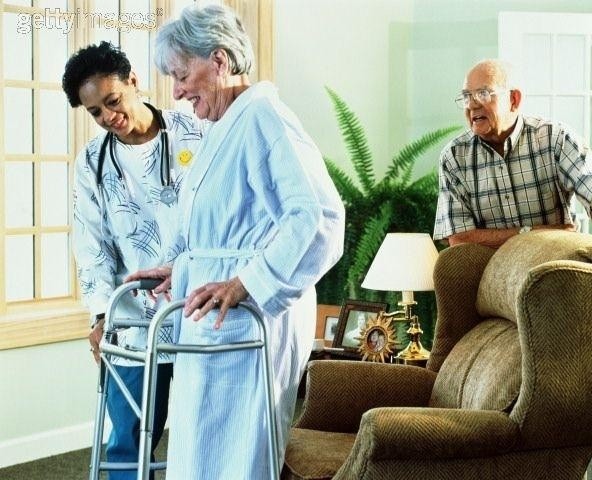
top-left (368, 330), bottom-right (379, 350)
top-left (123, 3), bottom-right (347, 480)
top-left (61, 40), bottom-right (205, 479)
top-left (374, 334), bottom-right (385, 352)
top-left (433, 60), bottom-right (592, 249)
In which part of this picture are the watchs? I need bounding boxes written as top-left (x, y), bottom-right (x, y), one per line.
top-left (519, 226), bottom-right (531, 234)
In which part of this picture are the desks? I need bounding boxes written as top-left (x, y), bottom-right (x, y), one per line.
top-left (297, 348), bottom-right (428, 397)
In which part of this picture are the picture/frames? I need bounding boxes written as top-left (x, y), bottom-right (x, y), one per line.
top-left (331, 300), bottom-right (390, 357)
top-left (356, 311), bottom-right (401, 365)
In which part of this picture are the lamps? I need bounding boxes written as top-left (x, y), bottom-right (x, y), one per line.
top-left (360, 232), bottom-right (439, 360)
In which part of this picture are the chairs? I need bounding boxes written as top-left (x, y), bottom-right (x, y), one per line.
top-left (282, 227), bottom-right (591, 479)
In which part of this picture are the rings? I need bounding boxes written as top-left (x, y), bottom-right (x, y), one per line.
top-left (212, 297), bottom-right (220, 306)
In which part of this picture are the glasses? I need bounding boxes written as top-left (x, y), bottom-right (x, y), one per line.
top-left (455, 89), bottom-right (504, 109)
